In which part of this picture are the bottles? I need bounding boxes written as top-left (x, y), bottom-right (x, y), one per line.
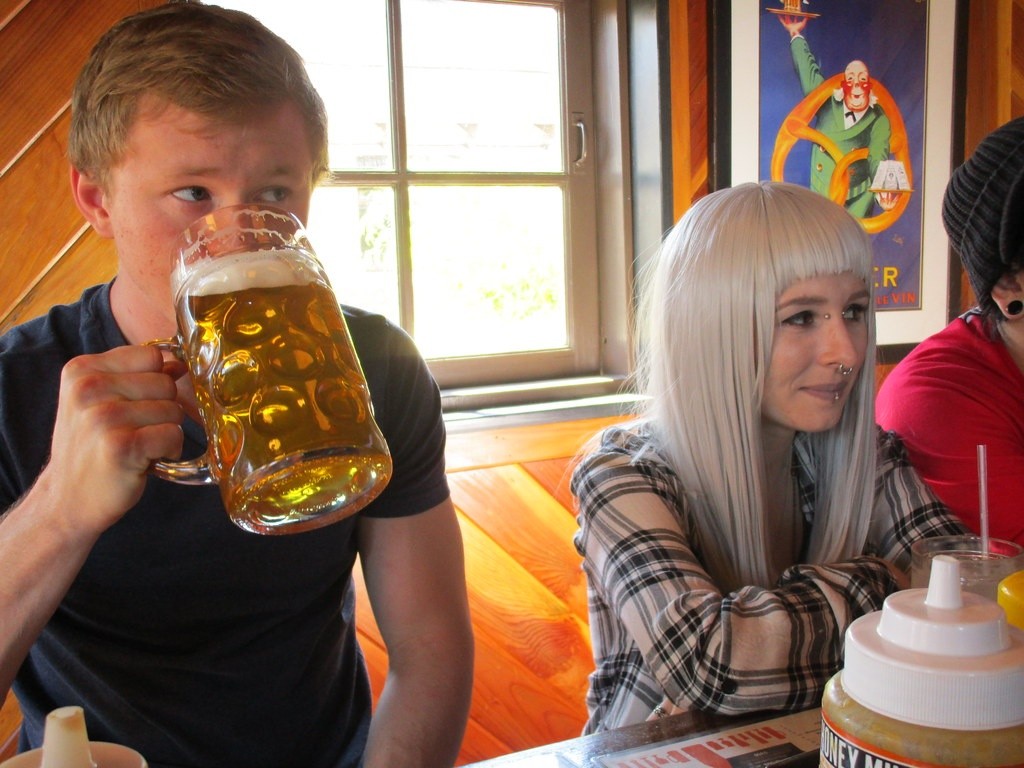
top-left (820, 555), bottom-right (1024, 768)
top-left (996, 571), bottom-right (1024, 636)
top-left (1, 706), bottom-right (148, 768)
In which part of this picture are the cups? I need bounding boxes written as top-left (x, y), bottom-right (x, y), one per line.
top-left (140, 204), bottom-right (392, 535)
top-left (911, 537), bottom-right (1024, 599)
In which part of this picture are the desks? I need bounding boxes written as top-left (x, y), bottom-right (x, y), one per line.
top-left (456, 710), bottom-right (820, 768)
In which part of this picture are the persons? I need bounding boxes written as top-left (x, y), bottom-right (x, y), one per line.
top-left (873, 116), bottom-right (1024, 556)
top-left (0, 0), bottom-right (475, 768)
top-left (558, 180), bottom-right (981, 738)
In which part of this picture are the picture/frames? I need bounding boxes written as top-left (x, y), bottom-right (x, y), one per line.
top-left (708, 1), bottom-right (966, 361)
